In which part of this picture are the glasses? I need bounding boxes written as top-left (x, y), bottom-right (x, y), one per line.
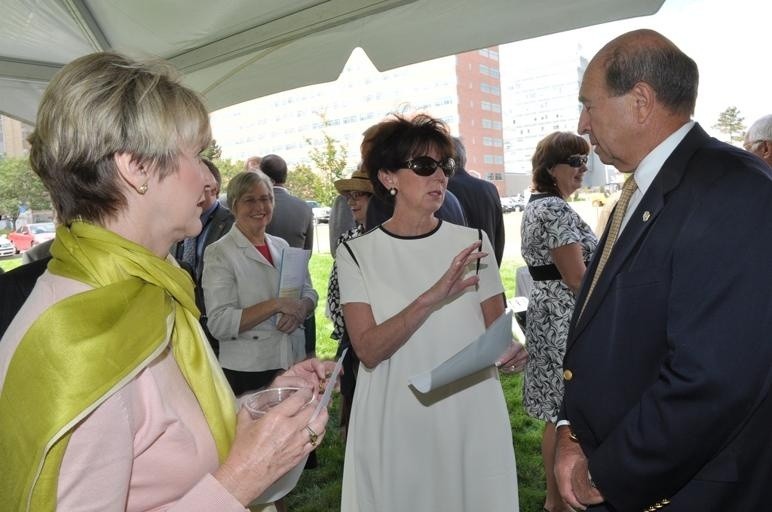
top-left (556, 156), bottom-right (588, 167)
top-left (342, 191), bottom-right (363, 201)
top-left (400, 157), bottom-right (457, 177)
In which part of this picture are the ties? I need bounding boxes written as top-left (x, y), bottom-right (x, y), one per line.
top-left (575, 174), bottom-right (637, 328)
top-left (182, 214), bottom-right (208, 271)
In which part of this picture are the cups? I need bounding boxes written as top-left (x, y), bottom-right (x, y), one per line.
top-left (246, 388), bottom-right (304, 419)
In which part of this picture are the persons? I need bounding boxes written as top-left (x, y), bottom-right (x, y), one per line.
top-left (0, 50), bottom-right (343, 511)
top-left (553, 26), bottom-right (772, 512)
top-left (327, 109), bottom-right (530, 512)
top-left (521, 131), bottom-right (600, 512)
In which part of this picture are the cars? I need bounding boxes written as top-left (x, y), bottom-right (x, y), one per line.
top-left (301, 200), bottom-right (334, 225)
top-left (0, 232), bottom-right (17, 256)
top-left (590, 183), bottom-right (621, 206)
top-left (8, 220), bottom-right (60, 251)
top-left (490, 191), bottom-right (532, 213)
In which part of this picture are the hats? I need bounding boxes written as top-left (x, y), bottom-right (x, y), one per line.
top-left (334, 171), bottom-right (374, 195)
